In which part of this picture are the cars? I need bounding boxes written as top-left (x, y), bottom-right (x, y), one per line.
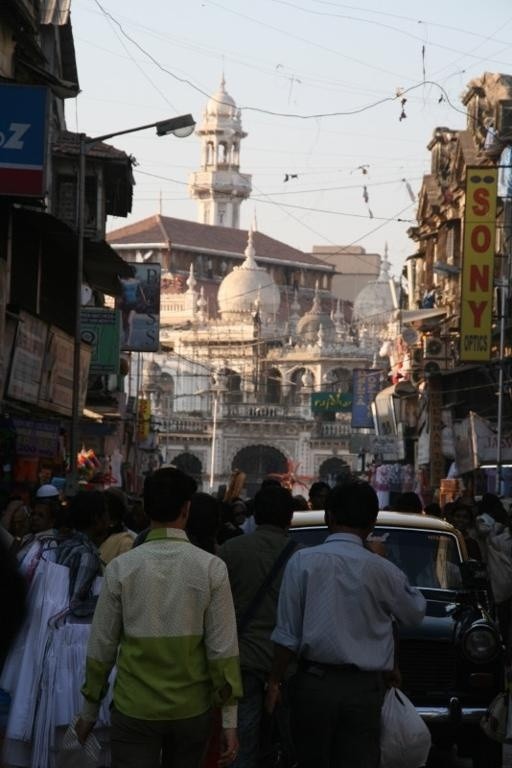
top-left (254, 502), bottom-right (508, 764)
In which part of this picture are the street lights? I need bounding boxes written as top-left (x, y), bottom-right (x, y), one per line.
top-left (65, 112), bottom-right (198, 494)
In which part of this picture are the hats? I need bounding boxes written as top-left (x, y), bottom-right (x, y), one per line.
top-left (36, 484), bottom-right (58, 497)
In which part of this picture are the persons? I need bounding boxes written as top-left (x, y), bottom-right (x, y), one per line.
top-left (1, 465), bottom-right (509, 767)
top-left (117, 265), bottom-right (149, 346)
